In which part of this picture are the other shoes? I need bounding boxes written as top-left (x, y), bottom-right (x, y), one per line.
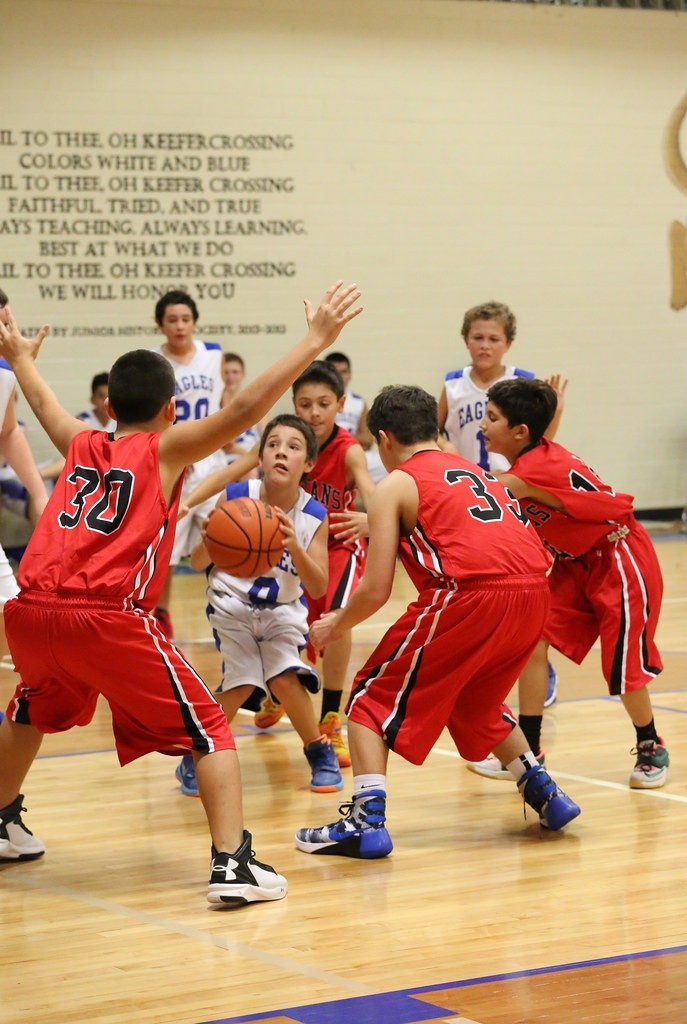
top-left (541, 662), bottom-right (558, 707)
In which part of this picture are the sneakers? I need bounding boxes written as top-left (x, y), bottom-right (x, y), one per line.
top-left (206, 830), bottom-right (290, 903)
top-left (319, 712), bottom-right (351, 766)
top-left (254, 692), bottom-right (285, 728)
top-left (174, 758), bottom-right (200, 797)
top-left (464, 751), bottom-right (547, 780)
top-left (296, 788), bottom-right (393, 858)
top-left (0, 794), bottom-right (47, 861)
top-left (628, 734), bottom-right (668, 788)
top-left (302, 735), bottom-right (344, 793)
top-left (515, 764), bottom-right (581, 828)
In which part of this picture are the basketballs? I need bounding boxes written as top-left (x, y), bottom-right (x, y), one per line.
top-left (206, 496), bottom-right (285, 579)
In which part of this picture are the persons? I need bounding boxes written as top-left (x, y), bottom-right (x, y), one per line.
top-left (0, 279), bottom-right (366, 908)
top-left (0, 288), bottom-right (672, 798)
top-left (293, 385), bottom-right (583, 861)
